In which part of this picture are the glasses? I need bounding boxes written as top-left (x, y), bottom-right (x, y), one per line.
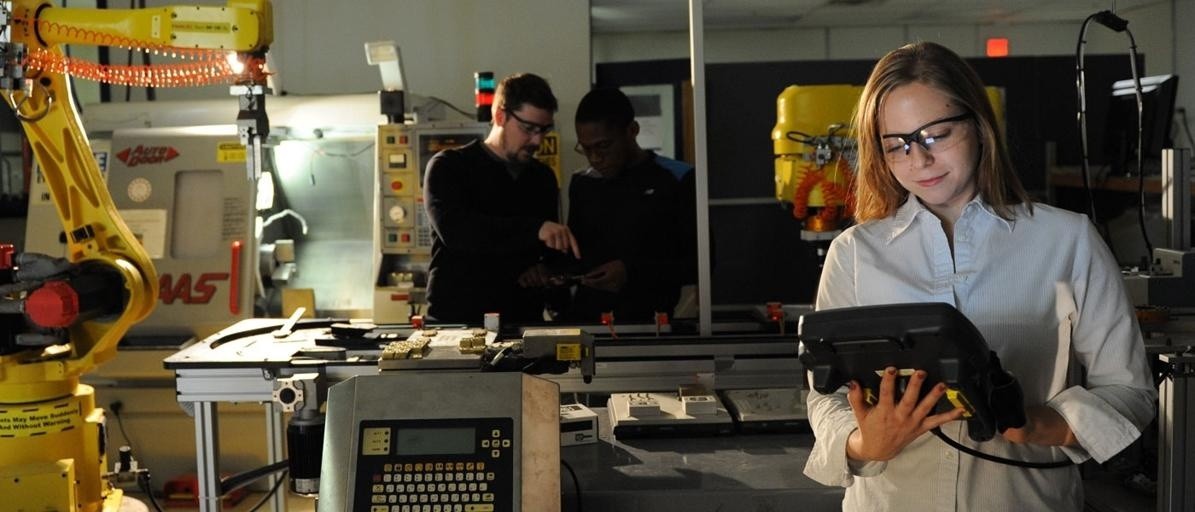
top-left (880, 114), bottom-right (975, 163)
top-left (504, 108), bottom-right (555, 135)
top-left (573, 134), bottom-right (620, 157)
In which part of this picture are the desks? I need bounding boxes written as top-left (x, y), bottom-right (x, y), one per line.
top-left (164, 316), bottom-right (846, 511)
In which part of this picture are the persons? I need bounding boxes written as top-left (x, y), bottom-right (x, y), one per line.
top-left (803, 41), bottom-right (1159, 512)
top-left (519, 88), bottom-right (698, 324)
top-left (423, 74), bottom-right (581, 323)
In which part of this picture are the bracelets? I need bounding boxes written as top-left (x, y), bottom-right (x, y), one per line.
top-left (1065, 428), bottom-right (1074, 447)
